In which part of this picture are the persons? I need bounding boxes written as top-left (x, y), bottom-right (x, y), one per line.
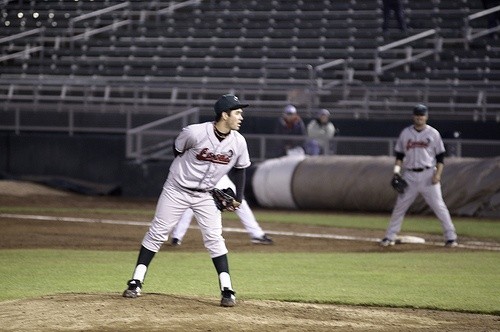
top-left (307, 109), bottom-right (334, 155)
top-left (122, 95), bottom-right (274, 307)
top-left (279, 105), bottom-right (307, 156)
top-left (378, 104), bottom-right (458, 248)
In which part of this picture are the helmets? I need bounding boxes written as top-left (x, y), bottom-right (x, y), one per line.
top-left (283, 105), bottom-right (298, 121)
top-left (317, 108), bottom-right (331, 125)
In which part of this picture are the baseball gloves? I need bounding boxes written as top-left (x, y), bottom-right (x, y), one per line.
top-left (205, 188), bottom-right (241, 213)
top-left (391, 173), bottom-right (408, 193)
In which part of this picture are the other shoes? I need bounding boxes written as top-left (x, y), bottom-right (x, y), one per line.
top-left (171, 238), bottom-right (181, 246)
top-left (445, 239), bottom-right (458, 248)
top-left (378, 237), bottom-right (396, 247)
top-left (250, 235), bottom-right (275, 245)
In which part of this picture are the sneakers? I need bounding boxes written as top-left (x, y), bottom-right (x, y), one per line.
top-left (123, 279), bottom-right (143, 298)
top-left (220, 287), bottom-right (236, 307)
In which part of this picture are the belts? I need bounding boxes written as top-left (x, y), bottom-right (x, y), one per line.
top-left (181, 187), bottom-right (210, 194)
top-left (407, 165), bottom-right (432, 173)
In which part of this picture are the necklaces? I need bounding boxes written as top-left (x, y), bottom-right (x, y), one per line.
top-left (214, 123), bottom-right (226, 139)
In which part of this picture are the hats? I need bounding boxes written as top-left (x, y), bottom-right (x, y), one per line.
top-left (215, 93), bottom-right (250, 117)
top-left (413, 104), bottom-right (428, 116)
